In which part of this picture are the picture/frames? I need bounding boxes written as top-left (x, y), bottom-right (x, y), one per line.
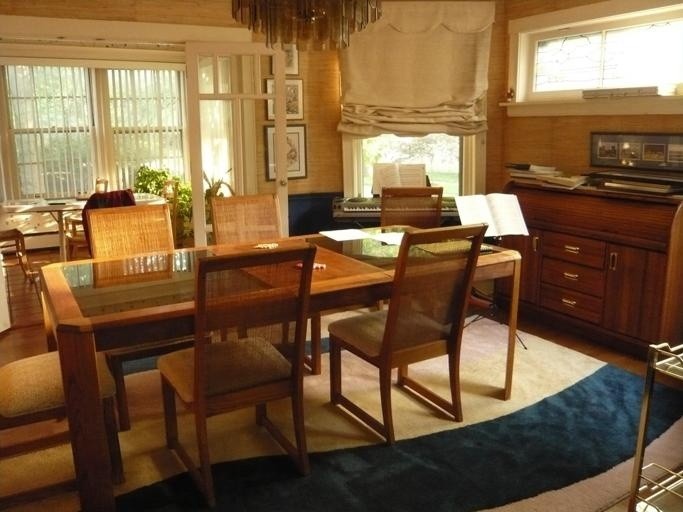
top-left (270, 42), bottom-right (300, 75)
top-left (264, 124), bottom-right (308, 182)
top-left (264, 76), bottom-right (304, 121)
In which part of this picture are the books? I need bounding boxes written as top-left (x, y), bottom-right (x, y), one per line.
top-left (455, 194), bottom-right (530, 237)
top-left (372, 160), bottom-right (426, 196)
top-left (506, 160), bottom-right (681, 194)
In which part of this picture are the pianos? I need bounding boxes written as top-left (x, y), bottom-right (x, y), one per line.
top-left (332, 198), bottom-right (458, 223)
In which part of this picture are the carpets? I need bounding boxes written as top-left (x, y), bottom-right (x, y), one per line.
top-left (0, 305), bottom-right (682, 510)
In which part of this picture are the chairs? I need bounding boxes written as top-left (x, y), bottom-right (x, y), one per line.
top-left (156, 245), bottom-right (316, 507)
top-left (327, 222), bottom-right (489, 446)
top-left (0, 348), bottom-right (124, 509)
top-left (0, 177), bottom-right (179, 280)
top-left (380, 186), bottom-right (444, 229)
top-left (84, 204), bottom-right (213, 425)
top-left (210, 193), bottom-right (325, 375)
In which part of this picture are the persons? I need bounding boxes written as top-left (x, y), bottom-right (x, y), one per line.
top-left (596, 139), bottom-right (682, 162)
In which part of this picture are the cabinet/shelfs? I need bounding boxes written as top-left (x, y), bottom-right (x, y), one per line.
top-left (542, 231), bottom-right (605, 340)
top-left (625, 334), bottom-right (681, 508)
top-left (605, 243), bottom-right (671, 360)
top-left (497, 229), bottom-right (540, 320)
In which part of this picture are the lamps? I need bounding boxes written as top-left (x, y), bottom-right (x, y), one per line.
top-left (230, 1), bottom-right (384, 52)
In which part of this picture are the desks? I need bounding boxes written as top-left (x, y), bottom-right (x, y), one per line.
top-left (45, 223), bottom-right (521, 512)
